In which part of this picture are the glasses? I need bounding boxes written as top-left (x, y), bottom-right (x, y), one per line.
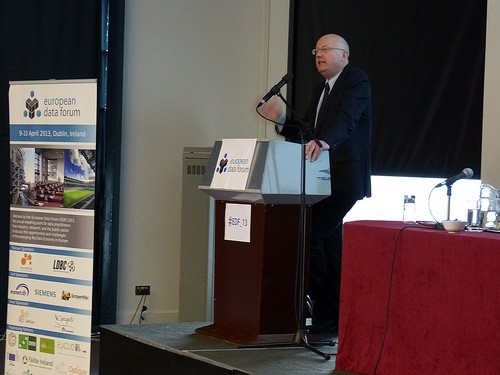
top-left (312, 46), bottom-right (345, 55)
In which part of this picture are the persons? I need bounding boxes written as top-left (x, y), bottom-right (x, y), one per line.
top-left (254, 34), bottom-right (372, 335)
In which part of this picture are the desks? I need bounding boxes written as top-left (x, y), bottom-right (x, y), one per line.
top-left (332, 218), bottom-right (500, 375)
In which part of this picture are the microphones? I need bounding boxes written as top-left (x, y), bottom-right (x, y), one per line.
top-left (257, 72), bottom-right (293, 105)
top-left (435, 168), bottom-right (474, 187)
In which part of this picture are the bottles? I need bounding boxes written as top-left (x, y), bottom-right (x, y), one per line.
top-left (403, 194), bottom-right (417, 225)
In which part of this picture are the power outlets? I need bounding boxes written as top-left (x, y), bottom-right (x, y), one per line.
top-left (135, 285), bottom-right (151, 296)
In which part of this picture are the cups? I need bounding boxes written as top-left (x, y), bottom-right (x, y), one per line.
top-left (468, 209), bottom-right (484, 230)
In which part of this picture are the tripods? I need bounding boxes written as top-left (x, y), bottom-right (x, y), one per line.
top-left (236, 91), bottom-right (337, 361)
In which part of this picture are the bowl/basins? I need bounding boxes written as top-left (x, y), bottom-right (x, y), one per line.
top-left (443, 221), bottom-right (467, 233)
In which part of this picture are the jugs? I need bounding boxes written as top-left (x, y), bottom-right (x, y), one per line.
top-left (480, 184), bottom-right (500, 232)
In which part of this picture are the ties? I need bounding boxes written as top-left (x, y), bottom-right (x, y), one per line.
top-left (316, 82), bottom-right (330, 136)
